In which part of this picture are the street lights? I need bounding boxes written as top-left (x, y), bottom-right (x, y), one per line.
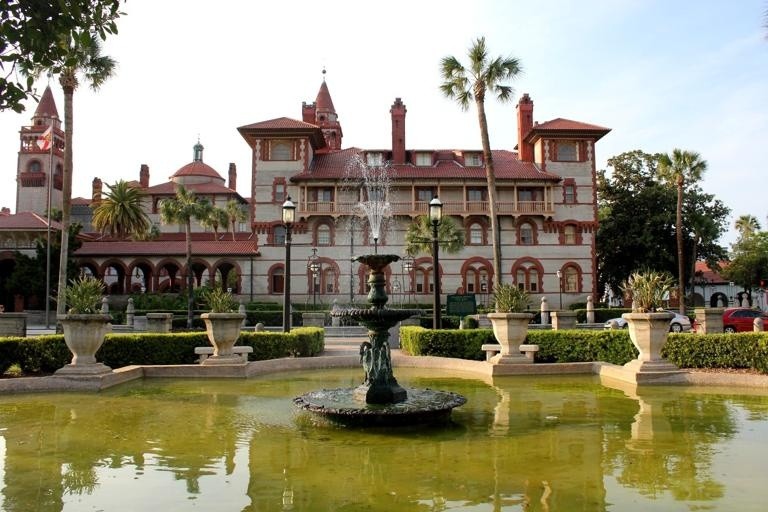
top-left (401, 246), bottom-right (416, 309)
top-left (728, 280), bottom-right (735, 305)
top-left (306, 246), bottom-right (321, 312)
top-left (557, 269), bottom-right (563, 310)
top-left (279, 193), bottom-right (296, 335)
top-left (425, 193), bottom-right (444, 330)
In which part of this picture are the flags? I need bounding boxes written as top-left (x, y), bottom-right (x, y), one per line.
top-left (36, 125), bottom-right (54, 151)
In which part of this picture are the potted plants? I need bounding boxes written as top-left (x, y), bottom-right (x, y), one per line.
top-left (49, 273), bottom-right (114, 365)
top-left (618, 266), bottom-right (676, 361)
top-left (200, 288), bottom-right (246, 357)
top-left (486, 282), bottom-right (535, 356)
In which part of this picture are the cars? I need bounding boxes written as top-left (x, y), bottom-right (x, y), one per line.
top-left (721, 306), bottom-right (768, 334)
top-left (603, 307), bottom-right (693, 335)
